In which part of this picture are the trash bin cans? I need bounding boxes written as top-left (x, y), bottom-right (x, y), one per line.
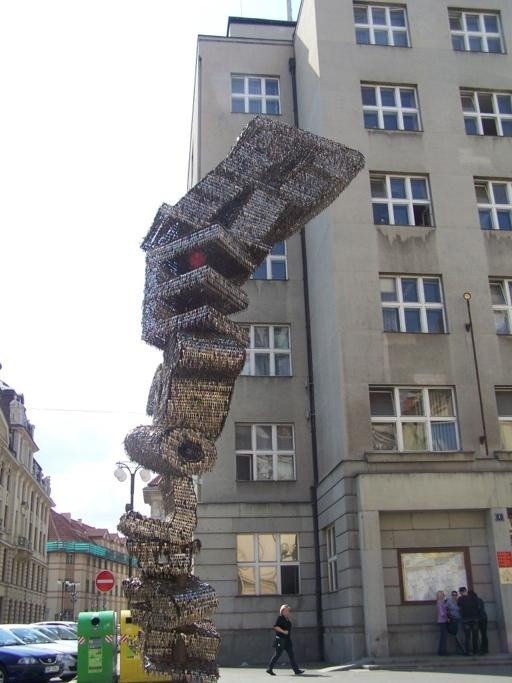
top-left (77, 609), bottom-right (118, 683)
top-left (120, 609), bottom-right (174, 683)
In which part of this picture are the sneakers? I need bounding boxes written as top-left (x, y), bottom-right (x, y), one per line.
top-left (266, 668), bottom-right (276, 675)
top-left (295, 670), bottom-right (304, 674)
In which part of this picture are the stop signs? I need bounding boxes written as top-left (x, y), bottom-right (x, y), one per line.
top-left (95, 570), bottom-right (114, 592)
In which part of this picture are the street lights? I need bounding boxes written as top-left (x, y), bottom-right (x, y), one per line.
top-left (114, 459), bottom-right (152, 581)
top-left (57, 578), bottom-right (69, 620)
top-left (69, 581), bottom-right (80, 620)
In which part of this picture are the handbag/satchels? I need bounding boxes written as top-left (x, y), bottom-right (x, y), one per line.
top-left (446, 619), bottom-right (457, 635)
top-left (273, 636), bottom-right (286, 649)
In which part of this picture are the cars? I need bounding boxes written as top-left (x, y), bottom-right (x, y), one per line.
top-left (0, 620), bottom-right (77, 682)
top-left (117, 624), bottom-right (120, 653)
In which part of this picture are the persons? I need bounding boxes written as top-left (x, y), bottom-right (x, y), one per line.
top-left (437, 586), bottom-right (488, 656)
top-left (266, 604), bottom-right (305, 675)
